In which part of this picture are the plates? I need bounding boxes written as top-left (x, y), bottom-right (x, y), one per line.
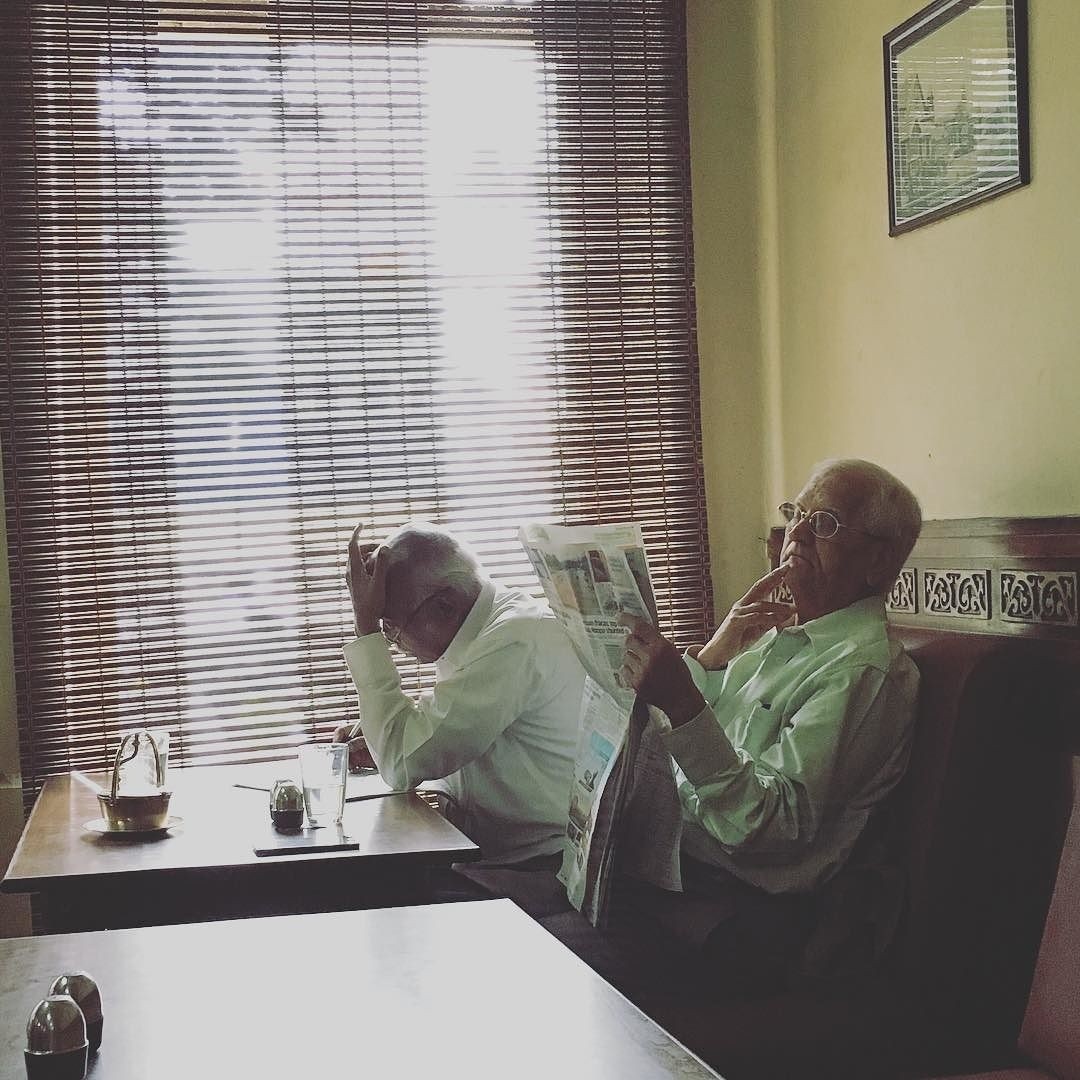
top-left (82, 814), bottom-right (183, 837)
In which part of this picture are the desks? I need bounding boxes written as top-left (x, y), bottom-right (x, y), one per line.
top-left (535, 645), bottom-right (703, 948)
top-left (0, 898), bottom-right (725, 1080)
top-left (0, 759), bottom-right (481, 935)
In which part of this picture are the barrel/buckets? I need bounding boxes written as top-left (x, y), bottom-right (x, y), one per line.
top-left (95, 730), bottom-right (172, 830)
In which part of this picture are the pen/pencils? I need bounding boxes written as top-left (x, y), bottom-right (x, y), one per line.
top-left (345, 720), bottom-right (361, 742)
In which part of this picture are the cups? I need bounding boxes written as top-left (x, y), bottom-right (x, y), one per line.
top-left (122, 731), bottom-right (170, 790)
top-left (296, 742), bottom-right (353, 826)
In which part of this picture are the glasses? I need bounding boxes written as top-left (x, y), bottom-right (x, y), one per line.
top-left (777, 501), bottom-right (894, 542)
top-left (381, 586), bottom-right (452, 644)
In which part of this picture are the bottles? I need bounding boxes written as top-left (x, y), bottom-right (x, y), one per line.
top-left (23, 972), bottom-right (104, 1080)
top-left (269, 778), bottom-right (304, 833)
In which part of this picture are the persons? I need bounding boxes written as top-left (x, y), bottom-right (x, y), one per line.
top-left (332, 521), bottom-right (587, 918)
top-left (535, 458), bottom-right (922, 1001)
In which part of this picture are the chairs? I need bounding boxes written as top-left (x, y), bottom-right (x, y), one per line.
top-left (637, 625), bottom-right (1080, 1080)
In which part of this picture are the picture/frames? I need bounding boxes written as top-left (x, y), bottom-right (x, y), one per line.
top-left (882, 0), bottom-right (1030, 236)
